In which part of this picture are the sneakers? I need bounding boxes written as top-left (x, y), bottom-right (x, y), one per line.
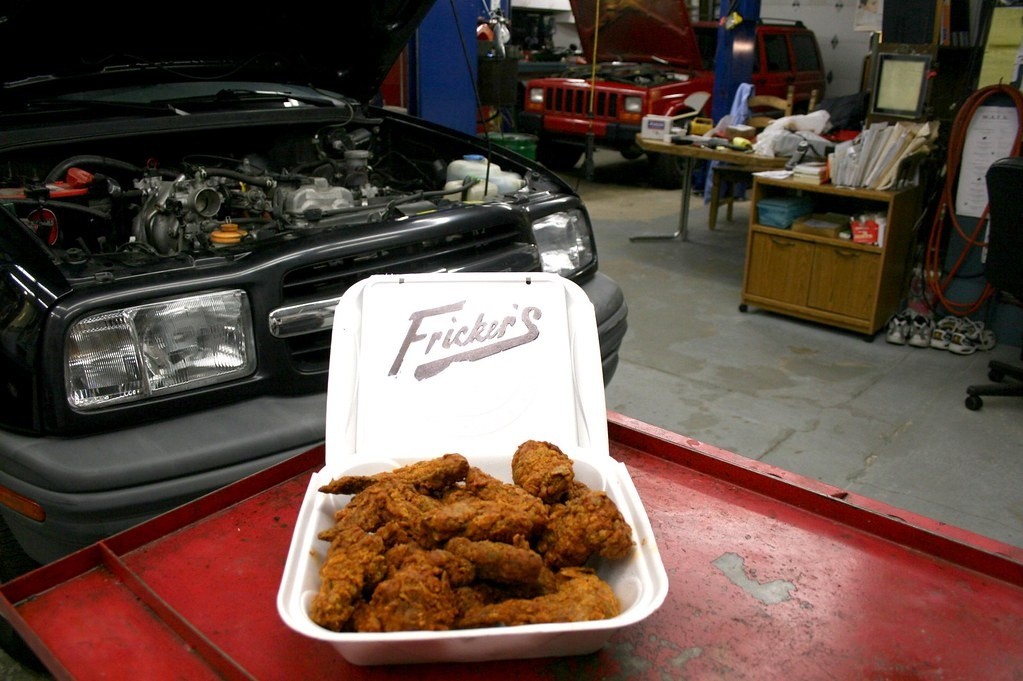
top-left (884, 313), bottom-right (909, 344)
top-left (910, 313), bottom-right (935, 347)
top-left (931, 316), bottom-right (966, 349)
top-left (950, 317), bottom-right (996, 354)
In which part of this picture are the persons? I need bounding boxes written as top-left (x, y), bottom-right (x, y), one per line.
top-left (858, 0), bottom-right (882, 26)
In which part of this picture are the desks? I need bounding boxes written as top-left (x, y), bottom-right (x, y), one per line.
top-left (632, 123), bottom-right (804, 233)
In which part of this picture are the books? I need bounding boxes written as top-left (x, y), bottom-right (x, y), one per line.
top-left (830, 119), bottom-right (940, 190)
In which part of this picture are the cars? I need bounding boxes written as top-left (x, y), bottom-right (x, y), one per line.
top-left (0, 1), bottom-right (633, 569)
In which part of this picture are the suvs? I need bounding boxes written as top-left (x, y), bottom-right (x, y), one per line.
top-left (521, 4), bottom-right (829, 182)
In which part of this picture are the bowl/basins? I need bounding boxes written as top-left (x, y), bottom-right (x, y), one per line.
top-left (271, 452), bottom-right (669, 667)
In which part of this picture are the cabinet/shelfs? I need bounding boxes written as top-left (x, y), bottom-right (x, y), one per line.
top-left (738, 160), bottom-right (922, 342)
